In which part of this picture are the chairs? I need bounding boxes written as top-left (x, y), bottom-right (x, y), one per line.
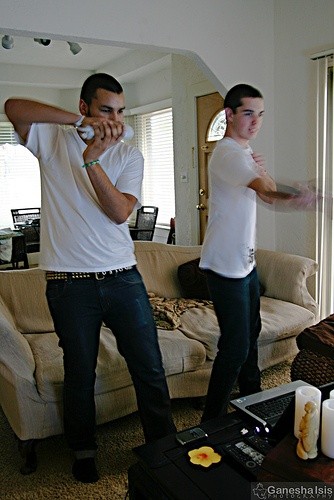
top-left (11, 207), bottom-right (40, 269)
top-left (133, 206), bottom-right (159, 241)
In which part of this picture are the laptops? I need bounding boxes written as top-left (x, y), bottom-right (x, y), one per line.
top-left (229, 379), bottom-right (333, 446)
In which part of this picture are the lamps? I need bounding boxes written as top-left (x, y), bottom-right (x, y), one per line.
top-left (67, 41), bottom-right (82, 54)
top-left (33, 38), bottom-right (52, 46)
top-left (2, 34), bottom-right (16, 49)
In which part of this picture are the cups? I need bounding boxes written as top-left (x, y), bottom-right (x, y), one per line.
top-left (293, 385), bottom-right (322, 439)
top-left (321, 399), bottom-right (334, 458)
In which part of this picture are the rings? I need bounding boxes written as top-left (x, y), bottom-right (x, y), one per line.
top-left (117, 136), bottom-right (124, 143)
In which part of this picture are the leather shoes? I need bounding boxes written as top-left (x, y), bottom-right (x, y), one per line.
top-left (44, 263), bottom-right (135, 283)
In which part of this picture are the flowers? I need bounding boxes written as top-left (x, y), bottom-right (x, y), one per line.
top-left (188, 446), bottom-right (221, 467)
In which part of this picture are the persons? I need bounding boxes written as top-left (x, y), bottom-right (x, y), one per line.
top-left (4, 73), bottom-right (178, 483)
top-left (296, 400), bottom-right (319, 460)
top-left (198, 84), bottom-right (322, 423)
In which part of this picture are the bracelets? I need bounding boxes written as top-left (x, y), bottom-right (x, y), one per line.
top-left (81, 159), bottom-right (99, 168)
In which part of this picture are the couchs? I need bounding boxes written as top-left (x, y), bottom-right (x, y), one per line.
top-left (290, 313), bottom-right (334, 386)
top-left (0, 240), bottom-right (320, 475)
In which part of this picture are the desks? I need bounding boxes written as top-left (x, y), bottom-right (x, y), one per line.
top-left (128, 383), bottom-right (334, 500)
